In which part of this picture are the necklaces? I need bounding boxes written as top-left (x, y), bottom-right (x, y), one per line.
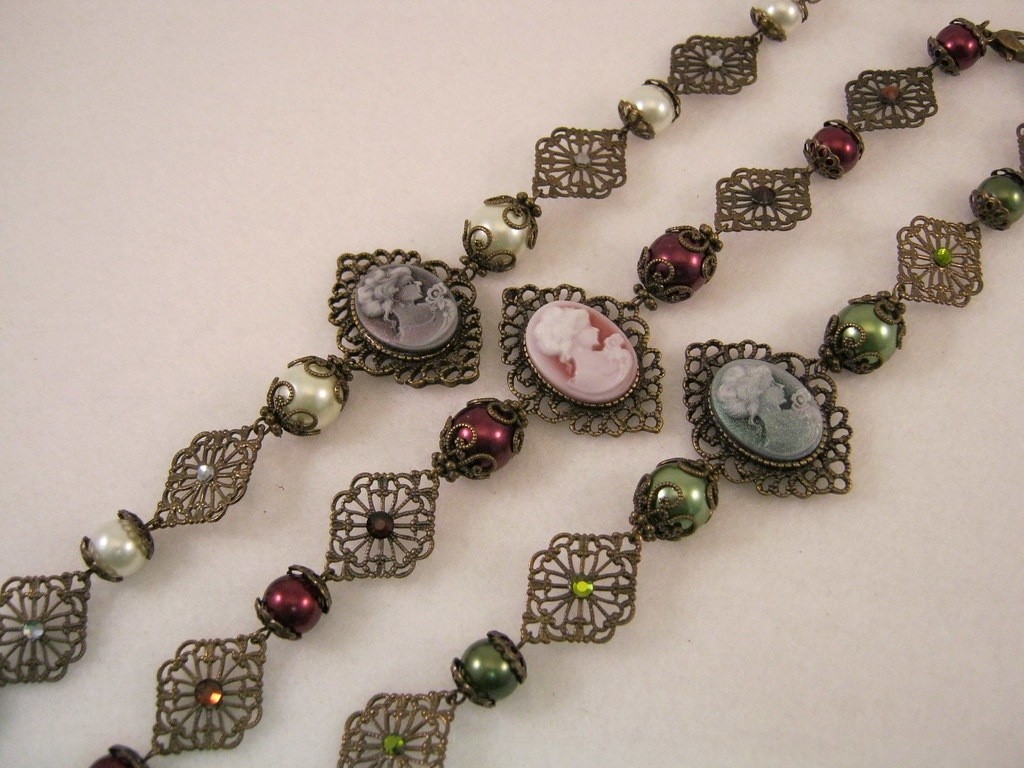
top-left (0, 1), bottom-right (1024, 768)
